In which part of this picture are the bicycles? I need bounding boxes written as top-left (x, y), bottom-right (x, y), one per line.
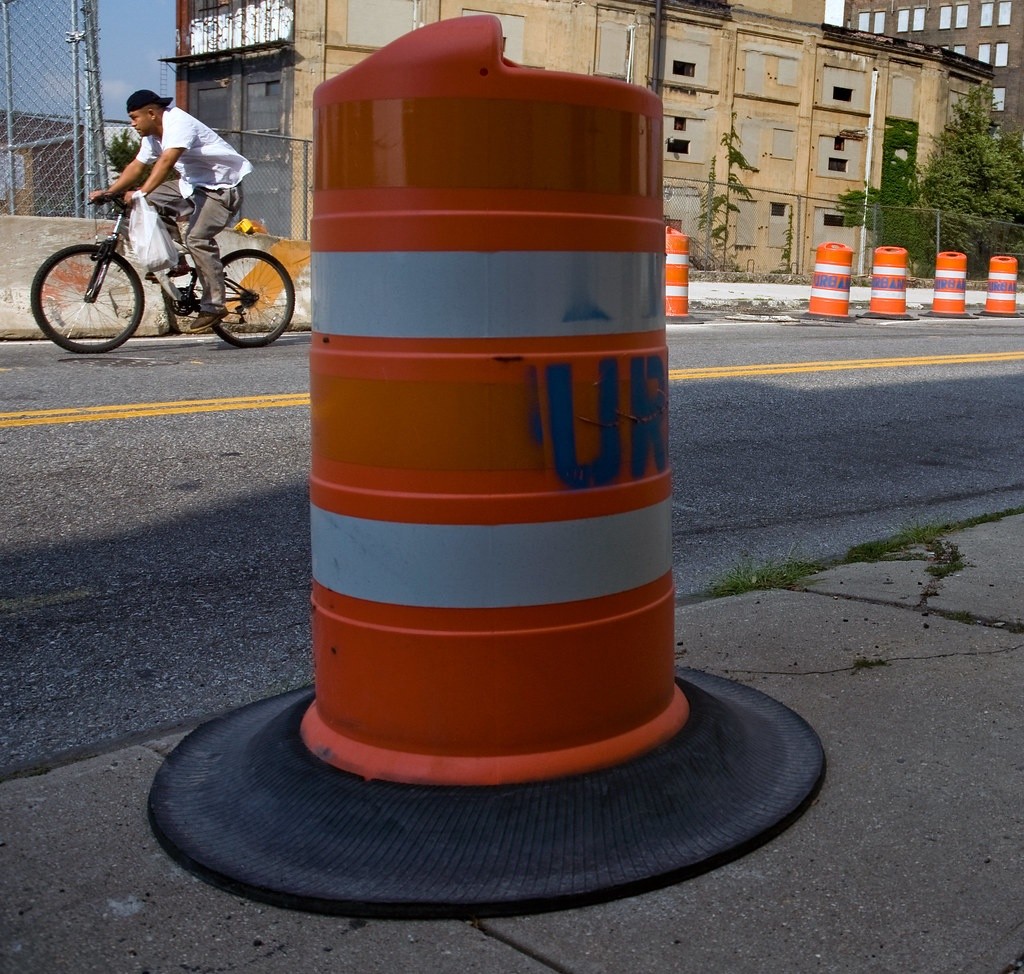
top-left (29, 192), bottom-right (296, 353)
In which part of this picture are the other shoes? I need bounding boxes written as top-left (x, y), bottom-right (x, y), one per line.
top-left (145, 256), bottom-right (189, 280)
top-left (189, 305), bottom-right (229, 332)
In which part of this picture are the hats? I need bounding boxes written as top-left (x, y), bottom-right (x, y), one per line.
top-left (126, 90), bottom-right (173, 113)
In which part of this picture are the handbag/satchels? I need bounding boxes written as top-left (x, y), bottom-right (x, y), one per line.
top-left (128, 190), bottom-right (179, 272)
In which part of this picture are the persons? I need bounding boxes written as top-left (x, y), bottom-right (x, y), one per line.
top-left (88, 89), bottom-right (255, 333)
